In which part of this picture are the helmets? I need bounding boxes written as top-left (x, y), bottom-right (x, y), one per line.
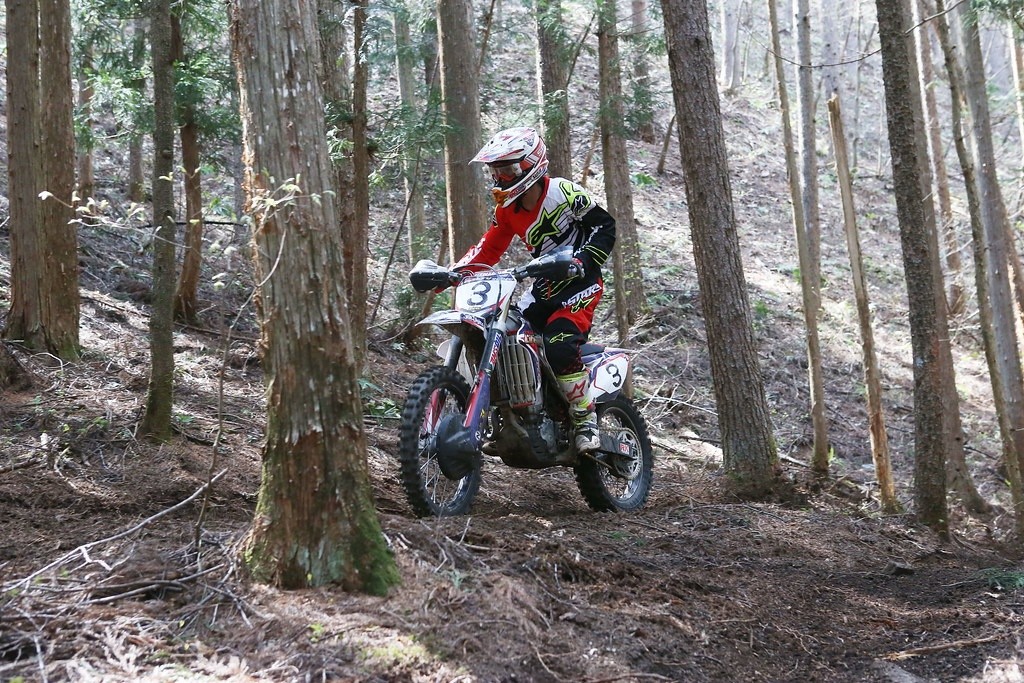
top-left (467, 127), bottom-right (549, 208)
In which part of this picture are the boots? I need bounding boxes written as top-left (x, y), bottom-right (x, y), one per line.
top-left (482, 441), bottom-right (501, 456)
top-left (556, 370), bottom-right (600, 453)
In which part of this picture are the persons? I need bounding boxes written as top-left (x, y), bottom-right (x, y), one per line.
top-left (435, 127), bottom-right (617, 455)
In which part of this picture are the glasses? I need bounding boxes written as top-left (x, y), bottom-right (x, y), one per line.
top-left (487, 136), bottom-right (545, 186)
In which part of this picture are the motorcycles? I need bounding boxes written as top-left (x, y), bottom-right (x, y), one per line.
top-left (396, 245), bottom-right (656, 520)
